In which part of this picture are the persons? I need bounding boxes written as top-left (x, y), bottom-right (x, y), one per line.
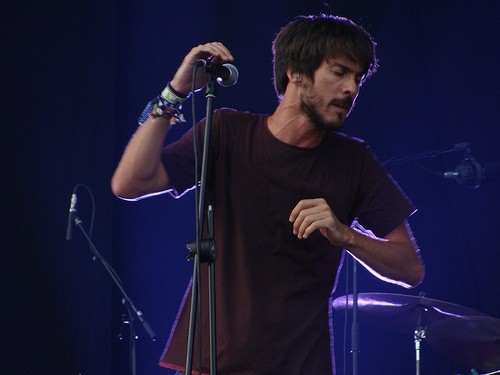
top-left (110, 14), bottom-right (426, 375)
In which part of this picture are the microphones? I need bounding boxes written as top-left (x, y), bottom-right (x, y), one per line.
top-left (67, 190), bottom-right (77, 240)
top-left (198, 59), bottom-right (239, 87)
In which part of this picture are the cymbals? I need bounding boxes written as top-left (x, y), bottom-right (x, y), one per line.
top-left (332, 291), bottom-right (499, 339)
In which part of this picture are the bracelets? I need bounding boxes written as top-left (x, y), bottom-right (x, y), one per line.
top-left (137, 80), bottom-right (188, 125)
